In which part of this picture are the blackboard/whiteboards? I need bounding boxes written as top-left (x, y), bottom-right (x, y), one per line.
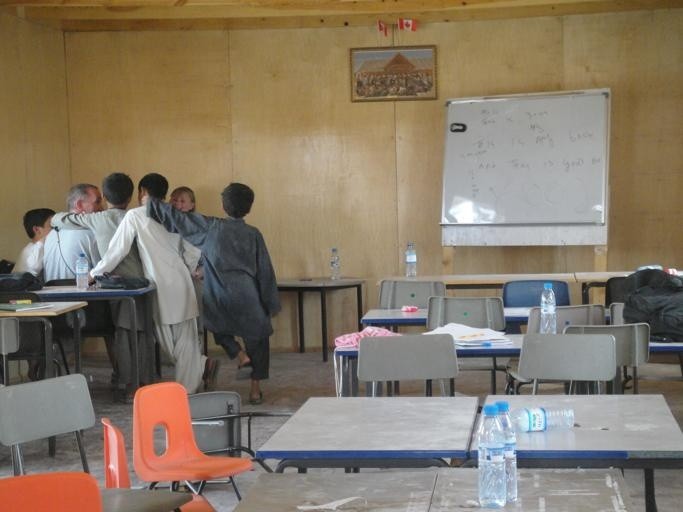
top-left (441, 226), bottom-right (608, 246)
top-left (439, 86), bottom-right (611, 225)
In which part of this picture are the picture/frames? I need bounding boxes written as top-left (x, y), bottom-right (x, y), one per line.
top-left (348, 44), bottom-right (439, 102)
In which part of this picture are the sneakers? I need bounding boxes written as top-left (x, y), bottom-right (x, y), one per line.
top-left (202, 359), bottom-right (221, 392)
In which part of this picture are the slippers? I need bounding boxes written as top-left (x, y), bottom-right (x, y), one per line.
top-left (238, 363), bottom-right (252, 369)
top-left (249, 391), bottom-right (262, 405)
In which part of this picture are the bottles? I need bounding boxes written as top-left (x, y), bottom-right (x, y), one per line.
top-left (634, 264), bottom-right (678, 278)
top-left (403, 242), bottom-right (417, 278)
top-left (507, 407), bottom-right (575, 434)
top-left (74, 253), bottom-right (89, 291)
top-left (476, 400), bottom-right (518, 508)
top-left (327, 248), bottom-right (340, 281)
top-left (537, 283), bottom-right (555, 335)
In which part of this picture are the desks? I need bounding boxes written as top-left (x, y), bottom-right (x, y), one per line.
top-left (0, 283), bottom-right (155, 456)
top-left (276, 275), bottom-right (366, 364)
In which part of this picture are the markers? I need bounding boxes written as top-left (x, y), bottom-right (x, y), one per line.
top-left (9, 299), bottom-right (32, 305)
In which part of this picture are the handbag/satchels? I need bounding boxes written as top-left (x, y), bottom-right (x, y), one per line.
top-left (94, 272), bottom-right (149, 289)
top-left (0, 272), bottom-right (42, 291)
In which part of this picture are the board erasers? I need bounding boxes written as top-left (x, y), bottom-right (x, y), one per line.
top-left (450, 123), bottom-right (467, 132)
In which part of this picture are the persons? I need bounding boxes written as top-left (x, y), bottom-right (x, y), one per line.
top-left (8, 170), bottom-right (223, 402)
top-left (138, 182), bottom-right (281, 406)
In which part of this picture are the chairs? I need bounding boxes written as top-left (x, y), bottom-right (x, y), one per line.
top-left (356, 277), bottom-right (654, 405)
